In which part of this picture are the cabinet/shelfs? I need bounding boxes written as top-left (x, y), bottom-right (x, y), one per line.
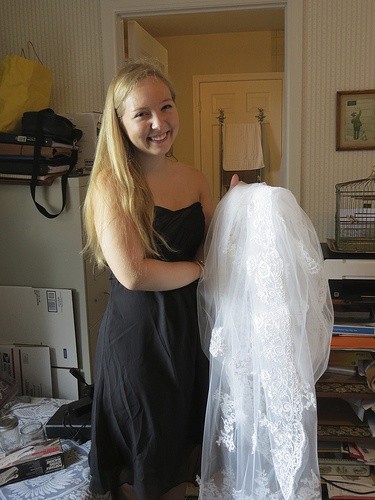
top-left (1, 177), bottom-right (112, 388)
top-left (315, 260), bottom-right (375, 500)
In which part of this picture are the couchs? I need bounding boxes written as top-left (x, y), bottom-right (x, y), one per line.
top-left (0, 397), bottom-right (110, 500)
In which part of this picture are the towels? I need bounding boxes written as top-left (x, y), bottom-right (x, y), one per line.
top-left (222, 121), bottom-right (265, 171)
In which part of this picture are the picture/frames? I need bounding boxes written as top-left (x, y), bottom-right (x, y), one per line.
top-left (336, 89), bottom-right (375, 151)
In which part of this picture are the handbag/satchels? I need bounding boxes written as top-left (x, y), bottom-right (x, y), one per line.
top-left (20, 108), bottom-right (83, 217)
top-left (0, 40), bottom-right (51, 134)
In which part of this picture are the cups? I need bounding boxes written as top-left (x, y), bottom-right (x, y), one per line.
top-left (19, 421), bottom-right (47, 448)
top-left (0, 414), bottom-right (23, 454)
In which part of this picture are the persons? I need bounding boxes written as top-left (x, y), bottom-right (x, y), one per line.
top-left (81, 62), bottom-right (240, 500)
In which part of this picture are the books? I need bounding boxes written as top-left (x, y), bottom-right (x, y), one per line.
top-left (329, 283), bottom-right (375, 350)
top-left (319, 440), bottom-right (375, 500)
top-left (0, 131), bottom-right (76, 187)
